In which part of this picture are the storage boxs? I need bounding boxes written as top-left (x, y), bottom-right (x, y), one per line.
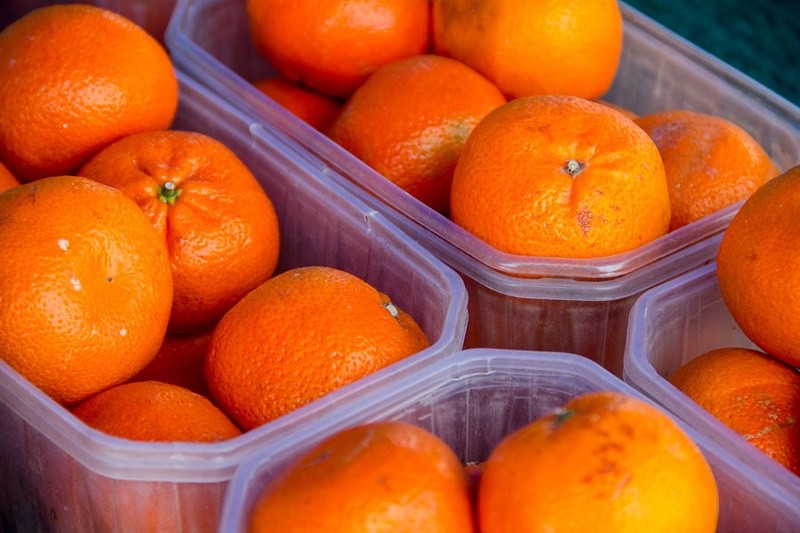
top-left (0, 0), bottom-right (800, 533)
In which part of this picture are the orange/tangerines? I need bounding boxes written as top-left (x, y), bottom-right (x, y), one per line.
top-left (0, 0), bottom-right (800, 533)
